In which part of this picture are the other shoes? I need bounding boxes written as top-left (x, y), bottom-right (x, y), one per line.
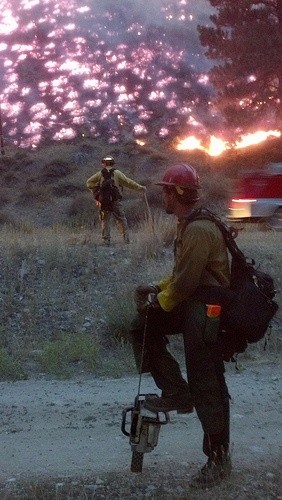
top-left (142, 390), bottom-right (194, 414)
top-left (125, 238), bottom-right (130, 244)
top-left (188, 451), bottom-right (233, 490)
top-left (103, 239), bottom-right (111, 245)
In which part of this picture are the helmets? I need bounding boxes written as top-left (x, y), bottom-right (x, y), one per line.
top-left (153, 162), bottom-right (203, 190)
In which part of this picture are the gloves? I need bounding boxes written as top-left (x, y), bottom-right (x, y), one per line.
top-left (133, 282), bottom-right (170, 322)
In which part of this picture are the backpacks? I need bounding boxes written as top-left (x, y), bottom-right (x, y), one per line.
top-left (94, 167), bottom-right (123, 204)
top-left (173, 206), bottom-right (280, 371)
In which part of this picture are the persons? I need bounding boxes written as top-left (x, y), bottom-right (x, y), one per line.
top-left (87, 154), bottom-right (148, 247)
top-left (129, 166), bottom-right (233, 487)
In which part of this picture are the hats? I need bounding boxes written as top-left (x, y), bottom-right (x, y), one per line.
top-left (103, 155), bottom-right (114, 161)
